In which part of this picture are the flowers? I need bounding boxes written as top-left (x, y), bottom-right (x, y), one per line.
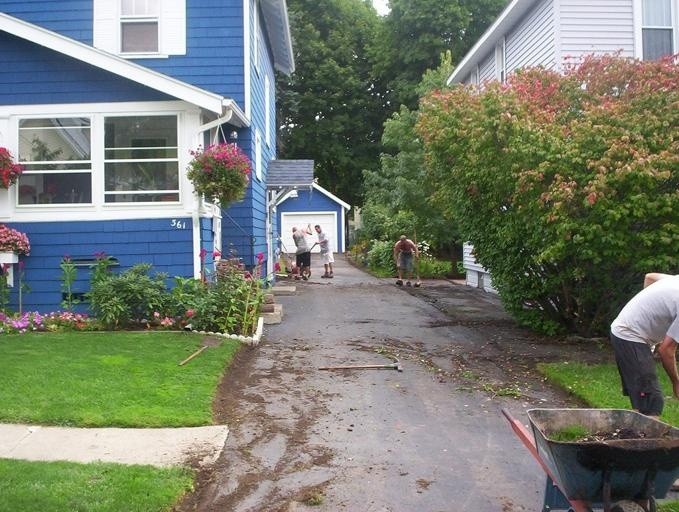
top-left (0, 223), bottom-right (31, 256)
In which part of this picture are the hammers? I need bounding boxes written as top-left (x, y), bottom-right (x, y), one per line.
top-left (318, 357), bottom-right (403, 371)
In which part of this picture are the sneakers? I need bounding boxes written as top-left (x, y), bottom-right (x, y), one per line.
top-left (321, 271), bottom-right (334, 277)
top-left (396, 279), bottom-right (412, 286)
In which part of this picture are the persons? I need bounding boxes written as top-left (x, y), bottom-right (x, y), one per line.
top-left (394, 234), bottom-right (419, 286)
top-left (293, 224), bottom-right (313, 269)
top-left (315, 225), bottom-right (334, 277)
top-left (611, 273), bottom-right (679, 421)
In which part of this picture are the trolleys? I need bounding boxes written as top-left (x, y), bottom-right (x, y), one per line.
top-left (287, 242), bottom-right (320, 279)
top-left (500, 402), bottom-right (678, 512)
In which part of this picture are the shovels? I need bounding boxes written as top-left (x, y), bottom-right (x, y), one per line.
top-left (178, 337), bottom-right (220, 366)
top-left (413, 229), bottom-right (421, 287)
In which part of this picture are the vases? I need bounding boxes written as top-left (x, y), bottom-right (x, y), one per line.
top-left (0, 251), bottom-right (18, 264)
